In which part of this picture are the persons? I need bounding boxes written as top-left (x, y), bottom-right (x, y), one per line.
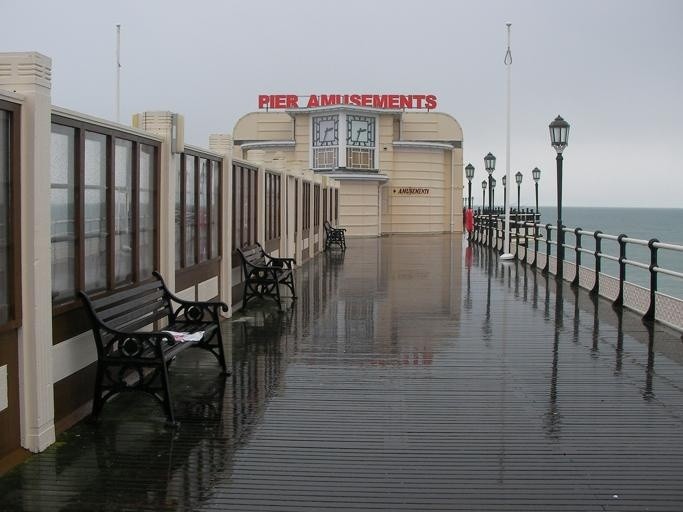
top-left (462, 206), bottom-right (466, 232)
top-left (471, 209), bottom-right (477, 220)
top-left (477, 206), bottom-right (481, 216)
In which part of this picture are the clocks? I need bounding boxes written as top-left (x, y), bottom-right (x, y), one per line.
top-left (313, 115), bottom-right (338, 145)
top-left (346, 115), bottom-right (375, 147)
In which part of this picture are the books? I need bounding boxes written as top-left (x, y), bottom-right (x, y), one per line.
top-left (157, 329), bottom-right (190, 343)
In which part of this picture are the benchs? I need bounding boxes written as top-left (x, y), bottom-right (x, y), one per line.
top-left (324, 221), bottom-right (346, 252)
top-left (77, 271), bottom-right (232, 429)
top-left (237, 241), bottom-right (297, 305)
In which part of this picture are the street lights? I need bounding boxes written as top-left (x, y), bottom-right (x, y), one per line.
top-left (532, 167), bottom-right (540, 214)
top-left (503, 18), bottom-right (513, 259)
top-left (484, 152), bottom-right (497, 248)
top-left (465, 163), bottom-right (475, 242)
top-left (516, 171), bottom-right (522, 212)
top-left (548, 115), bottom-right (569, 279)
top-left (482, 175), bottom-right (509, 207)
